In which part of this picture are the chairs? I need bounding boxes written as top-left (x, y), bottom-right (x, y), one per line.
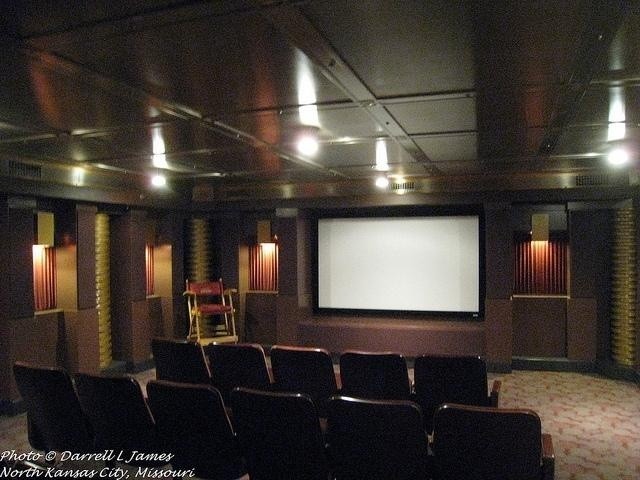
top-left (183, 277), bottom-right (239, 345)
